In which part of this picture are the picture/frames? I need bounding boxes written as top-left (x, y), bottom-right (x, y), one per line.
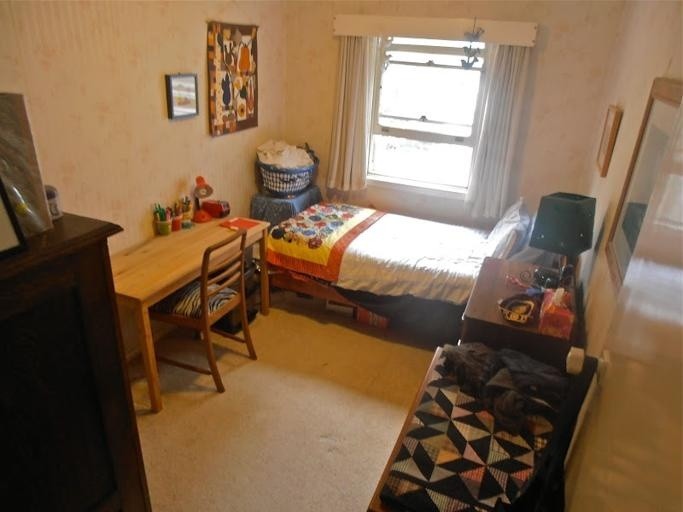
top-left (596, 104), bottom-right (624, 178)
top-left (163, 72), bottom-right (200, 120)
top-left (207, 19), bottom-right (261, 136)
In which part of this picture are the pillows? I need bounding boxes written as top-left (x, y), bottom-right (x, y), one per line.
top-left (487, 195), bottom-right (535, 260)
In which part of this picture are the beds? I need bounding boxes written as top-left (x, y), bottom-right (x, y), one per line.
top-left (269, 198), bottom-right (554, 325)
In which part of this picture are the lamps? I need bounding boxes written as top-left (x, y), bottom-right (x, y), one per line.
top-left (532, 190), bottom-right (596, 289)
top-left (193, 176), bottom-right (212, 223)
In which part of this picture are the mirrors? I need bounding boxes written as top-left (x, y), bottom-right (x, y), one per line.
top-left (604, 78), bottom-right (682, 295)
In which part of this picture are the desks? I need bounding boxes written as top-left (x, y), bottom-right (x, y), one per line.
top-left (251, 184), bottom-right (320, 222)
top-left (0, 213), bottom-right (153, 511)
top-left (365, 347), bottom-right (570, 510)
top-left (107, 217), bottom-right (272, 414)
top-left (463, 251), bottom-right (585, 352)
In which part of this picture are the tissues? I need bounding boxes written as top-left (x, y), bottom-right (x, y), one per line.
top-left (538, 285), bottom-right (577, 341)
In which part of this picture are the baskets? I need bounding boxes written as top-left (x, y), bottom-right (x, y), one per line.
top-left (258, 146), bottom-right (319, 199)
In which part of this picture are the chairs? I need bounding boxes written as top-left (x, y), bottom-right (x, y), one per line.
top-left (147, 228), bottom-right (258, 393)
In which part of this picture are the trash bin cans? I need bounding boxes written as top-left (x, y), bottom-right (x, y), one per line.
top-left (203, 263), bottom-right (261, 333)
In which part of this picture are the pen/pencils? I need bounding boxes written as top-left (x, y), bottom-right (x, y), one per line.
top-left (153, 196), bottom-right (192, 221)
